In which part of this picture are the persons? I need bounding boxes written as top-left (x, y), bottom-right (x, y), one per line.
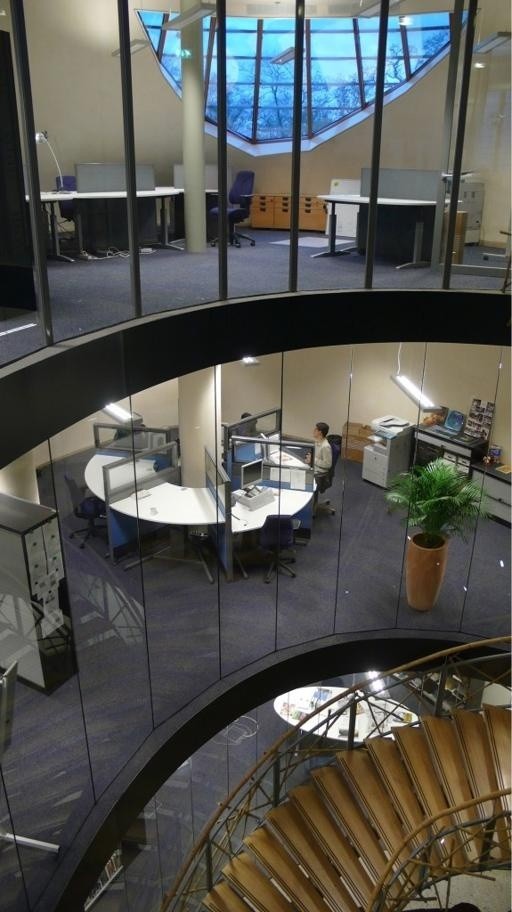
top-left (240, 412), bottom-right (258, 435)
top-left (304, 422), bottom-right (333, 501)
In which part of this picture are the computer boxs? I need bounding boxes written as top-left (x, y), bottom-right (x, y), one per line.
top-left (439, 210), bottom-right (468, 264)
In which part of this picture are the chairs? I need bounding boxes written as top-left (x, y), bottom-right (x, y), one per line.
top-left (209, 171), bottom-right (255, 249)
top-left (312, 435), bottom-right (342, 519)
top-left (65, 474), bottom-right (108, 549)
top-left (56, 175), bottom-right (76, 220)
top-left (255, 515), bottom-right (296, 584)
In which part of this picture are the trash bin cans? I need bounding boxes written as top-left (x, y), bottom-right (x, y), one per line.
top-left (328, 434), bottom-right (341, 455)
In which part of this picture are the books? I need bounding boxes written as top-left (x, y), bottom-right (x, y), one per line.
top-left (81, 849), bottom-right (124, 909)
top-left (488, 443), bottom-right (512, 475)
top-left (281, 671), bottom-right (470, 738)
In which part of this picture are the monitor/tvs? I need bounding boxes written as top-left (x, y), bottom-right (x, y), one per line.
top-left (260, 433), bottom-right (269, 461)
top-left (241, 459), bottom-right (263, 498)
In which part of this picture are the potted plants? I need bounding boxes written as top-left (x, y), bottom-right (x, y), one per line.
top-left (382, 456), bottom-right (493, 613)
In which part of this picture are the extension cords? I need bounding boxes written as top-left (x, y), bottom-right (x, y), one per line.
top-left (96, 249), bottom-right (112, 257)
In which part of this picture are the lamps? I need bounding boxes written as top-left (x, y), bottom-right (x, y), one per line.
top-left (102, 400), bottom-right (143, 427)
top-left (390, 343), bottom-right (444, 412)
top-left (35, 128), bottom-right (64, 186)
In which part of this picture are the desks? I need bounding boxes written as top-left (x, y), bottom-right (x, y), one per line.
top-left (24, 188), bottom-right (220, 263)
top-left (310, 169), bottom-right (462, 271)
top-left (84, 437), bottom-right (316, 533)
top-left (274, 686), bottom-right (420, 758)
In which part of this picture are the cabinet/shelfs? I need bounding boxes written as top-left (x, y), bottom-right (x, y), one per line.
top-left (250, 194), bottom-right (326, 232)
top-left (389, 669), bottom-right (485, 715)
top-left (361, 415), bottom-right (511, 523)
top-left (448, 180), bottom-right (485, 246)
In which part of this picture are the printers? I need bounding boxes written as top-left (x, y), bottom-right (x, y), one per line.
top-left (362, 415), bottom-right (414, 489)
